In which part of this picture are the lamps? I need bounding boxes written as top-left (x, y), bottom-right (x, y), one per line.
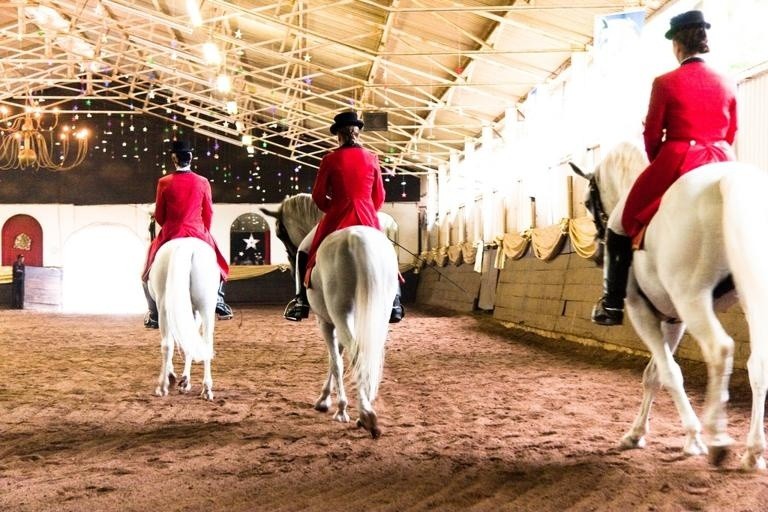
top-left (0, 77), bottom-right (90, 172)
top-left (186, 2), bottom-right (258, 155)
top-left (25, 0), bottom-right (105, 73)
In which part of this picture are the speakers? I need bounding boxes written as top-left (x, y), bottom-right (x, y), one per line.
top-left (379, 174), bottom-right (421, 203)
top-left (364, 112), bottom-right (388, 132)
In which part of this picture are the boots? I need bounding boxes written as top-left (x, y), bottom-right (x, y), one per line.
top-left (144, 283), bottom-right (160, 329)
top-left (390, 295), bottom-right (402, 324)
top-left (284, 250), bottom-right (310, 318)
top-left (592, 227), bottom-right (633, 326)
top-left (216, 277), bottom-right (233, 321)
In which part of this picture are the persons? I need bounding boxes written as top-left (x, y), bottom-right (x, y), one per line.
top-left (588, 8), bottom-right (740, 327)
top-left (11, 251), bottom-right (25, 307)
top-left (284, 109), bottom-right (407, 325)
top-left (141, 137), bottom-right (234, 330)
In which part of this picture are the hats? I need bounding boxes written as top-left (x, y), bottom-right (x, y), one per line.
top-left (167, 139), bottom-right (196, 152)
top-left (664, 9), bottom-right (712, 40)
top-left (329, 111), bottom-right (364, 135)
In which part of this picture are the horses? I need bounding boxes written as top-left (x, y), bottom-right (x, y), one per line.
top-left (259, 194), bottom-right (400, 440)
top-left (145, 210), bottom-right (221, 401)
top-left (566, 140), bottom-right (768, 473)
